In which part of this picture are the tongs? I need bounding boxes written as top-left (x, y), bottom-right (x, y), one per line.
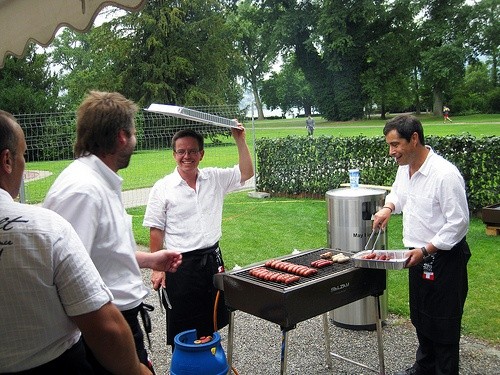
top-left (365, 221), bottom-right (382, 254)
top-left (159, 285), bottom-right (174, 310)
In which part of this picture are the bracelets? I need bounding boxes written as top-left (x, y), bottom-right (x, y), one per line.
top-left (383, 206), bottom-right (392, 213)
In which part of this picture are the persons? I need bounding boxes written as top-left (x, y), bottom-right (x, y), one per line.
top-left (443, 105), bottom-right (453, 123)
top-left (306, 116), bottom-right (315, 137)
top-left (41, 88), bottom-right (182, 375)
top-left (0, 110), bottom-right (153, 375)
top-left (373, 114), bottom-right (472, 375)
top-left (142, 118), bottom-right (254, 353)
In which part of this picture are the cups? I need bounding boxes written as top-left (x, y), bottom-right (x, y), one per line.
top-left (349, 169), bottom-right (359, 189)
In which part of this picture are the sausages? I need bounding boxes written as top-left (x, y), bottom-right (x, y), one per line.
top-left (248, 268), bottom-right (301, 284)
top-left (311, 259), bottom-right (333, 268)
top-left (362, 253), bottom-right (387, 261)
top-left (321, 252), bottom-right (350, 263)
top-left (265, 258), bottom-right (318, 276)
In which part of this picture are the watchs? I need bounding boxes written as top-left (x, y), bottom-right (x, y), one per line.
top-left (421, 246), bottom-right (435, 264)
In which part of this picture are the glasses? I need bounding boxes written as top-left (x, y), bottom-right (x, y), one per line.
top-left (174, 148), bottom-right (201, 156)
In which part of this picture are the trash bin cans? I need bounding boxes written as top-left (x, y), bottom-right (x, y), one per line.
top-left (324, 187), bottom-right (388, 329)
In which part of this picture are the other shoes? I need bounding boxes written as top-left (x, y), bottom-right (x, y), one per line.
top-left (404, 366), bottom-right (421, 375)
